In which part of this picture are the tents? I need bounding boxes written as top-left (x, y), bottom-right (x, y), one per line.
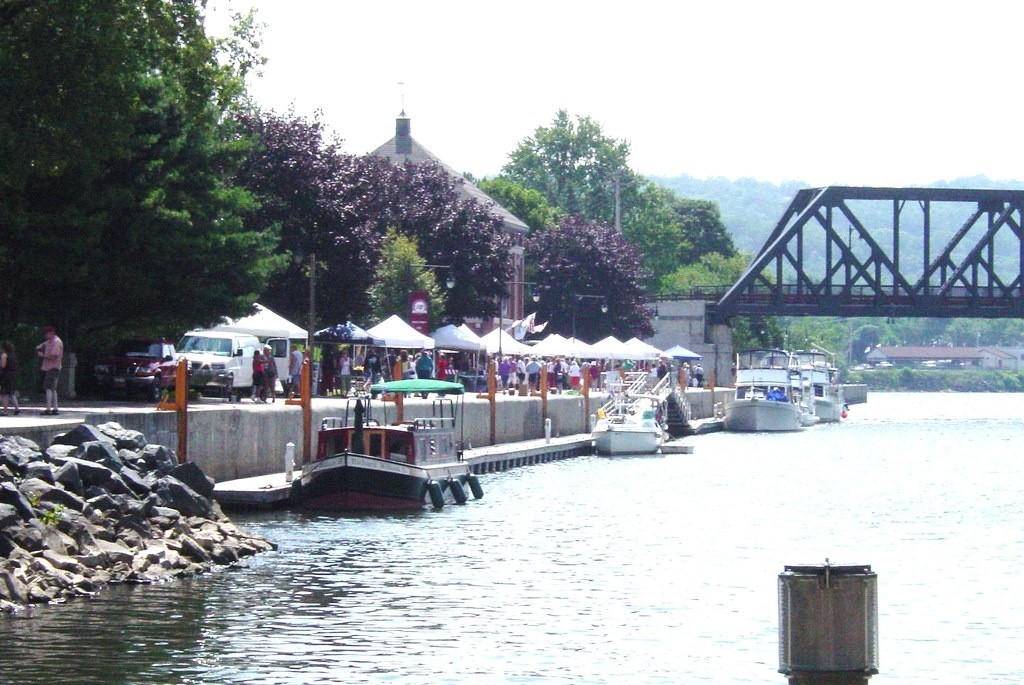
top-left (367, 314), bottom-right (435, 360)
top-left (481, 327), bottom-right (669, 368)
top-left (664, 344), bottom-right (703, 361)
top-left (313, 320), bottom-right (393, 381)
top-left (428, 323), bottom-right (487, 368)
top-left (209, 303), bottom-right (308, 347)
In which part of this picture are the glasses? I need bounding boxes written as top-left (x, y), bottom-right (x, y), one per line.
top-left (342, 352), bottom-right (347, 354)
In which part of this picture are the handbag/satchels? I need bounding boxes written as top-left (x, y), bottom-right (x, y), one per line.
top-left (557, 371), bottom-right (563, 381)
top-left (275, 377), bottom-right (284, 394)
top-left (409, 371), bottom-right (415, 375)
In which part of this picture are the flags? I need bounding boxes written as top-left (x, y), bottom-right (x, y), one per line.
top-left (513, 313), bottom-right (548, 340)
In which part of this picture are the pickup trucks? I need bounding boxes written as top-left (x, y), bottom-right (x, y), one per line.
top-left (93, 335), bottom-right (191, 402)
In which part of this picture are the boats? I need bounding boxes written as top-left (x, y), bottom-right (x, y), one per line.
top-left (725, 345), bottom-right (850, 431)
top-left (302, 376), bottom-right (471, 511)
top-left (591, 394), bottom-right (665, 455)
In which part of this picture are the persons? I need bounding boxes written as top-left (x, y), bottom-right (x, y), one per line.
top-left (414, 350), bottom-right (434, 399)
top-left (681, 362), bottom-right (704, 389)
top-left (649, 360), bottom-right (666, 380)
top-left (36, 326), bottom-right (63, 416)
top-left (249, 345), bottom-right (278, 404)
top-left (383, 349), bottom-right (397, 376)
top-left (0, 335), bottom-right (20, 417)
top-left (339, 350), bottom-right (353, 399)
top-left (435, 353), bottom-right (448, 398)
top-left (283, 343), bottom-right (301, 397)
top-left (399, 350), bottom-right (412, 399)
top-left (364, 346), bottom-right (382, 384)
top-left (489, 354), bottom-right (638, 395)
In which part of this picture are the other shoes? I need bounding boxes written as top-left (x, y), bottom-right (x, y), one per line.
top-left (0, 410), bottom-right (8, 417)
top-left (250, 394), bottom-right (255, 402)
top-left (262, 400), bottom-right (267, 403)
top-left (40, 409), bottom-right (50, 415)
top-left (14, 409), bottom-right (20, 415)
top-left (256, 399), bottom-right (265, 403)
top-left (50, 410), bottom-right (59, 415)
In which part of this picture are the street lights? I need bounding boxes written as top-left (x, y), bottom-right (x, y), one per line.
top-left (498, 280), bottom-right (541, 364)
top-left (567, 291), bottom-right (608, 340)
top-left (848, 224), bottom-right (864, 251)
top-left (407, 257), bottom-right (456, 333)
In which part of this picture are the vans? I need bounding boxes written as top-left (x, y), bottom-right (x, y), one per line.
top-left (174, 328), bottom-right (291, 404)
